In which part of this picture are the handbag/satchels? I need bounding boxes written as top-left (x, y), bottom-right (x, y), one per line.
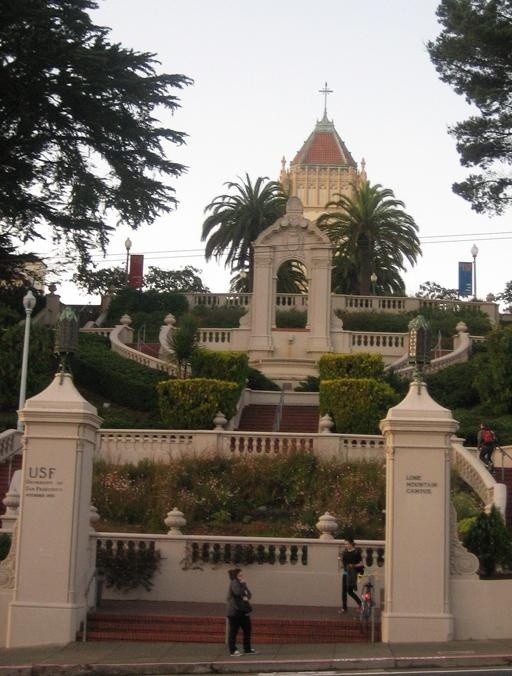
top-left (482, 430), bottom-right (494, 444)
top-left (234, 601), bottom-right (252, 613)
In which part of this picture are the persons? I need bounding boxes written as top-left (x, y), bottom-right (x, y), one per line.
top-left (359, 583), bottom-right (374, 633)
top-left (225, 567), bottom-right (260, 657)
top-left (477, 420), bottom-right (498, 476)
top-left (337, 535), bottom-right (366, 614)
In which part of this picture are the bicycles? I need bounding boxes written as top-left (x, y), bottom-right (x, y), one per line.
top-left (356, 570), bottom-right (379, 634)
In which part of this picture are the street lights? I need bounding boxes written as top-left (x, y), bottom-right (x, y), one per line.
top-left (15, 291), bottom-right (37, 431)
top-left (240, 266), bottom-right (247, 292)
top-left (124, 237), bottom-right (133, 276)
top-left (472, 244), bottom-right (480, 301)
top-left (369, 272), bottom-right (379, 296)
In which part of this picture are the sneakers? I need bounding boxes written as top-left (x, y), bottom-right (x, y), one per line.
top-left (230, 650), bottom-right (243, 656)
top-left (245, 649), bottom-right (256, 654)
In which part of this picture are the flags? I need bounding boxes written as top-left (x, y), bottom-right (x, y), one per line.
top-left (459, 261), bottom-right (473, 295)
top-left (129, 254), bottom-right (144, 288)
